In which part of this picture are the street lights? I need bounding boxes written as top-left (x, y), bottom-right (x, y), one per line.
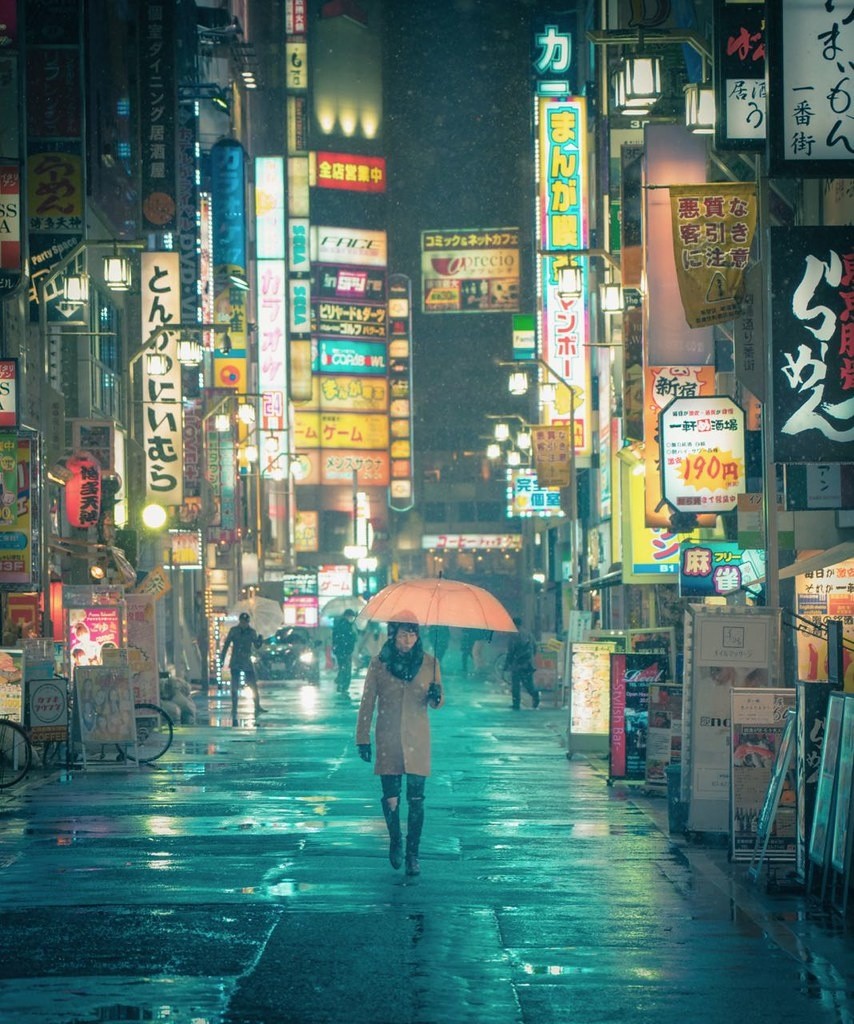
top-left (127, 320), bottom-right (233, 442)
top-left (260, 452), bottom-right (310, 538)
top-left (200, 393), bottom-right (266, 480)
top-left (32, 237), bottom-right (153, 639)
top-left (587, 26), bottom-right (779, 610)
top-left (534, 244), bottom-right (644, 319)
top-left (495, 358), bottom-right (580, 609)
top-left (230, 426), bottom-right (290, 518)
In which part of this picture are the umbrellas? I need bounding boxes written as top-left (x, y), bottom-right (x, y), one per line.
top-left (320, 593), bottom-right (364, 621)
top-left (227, 594), bottom-right (287, 642)
top-left (356, 568), bottom-right (518, 682)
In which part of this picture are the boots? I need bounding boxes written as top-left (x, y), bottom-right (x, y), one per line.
top-left (381, 797), bottom-right (402, 869)
top-left (404, 799), bottom-right (425, 875)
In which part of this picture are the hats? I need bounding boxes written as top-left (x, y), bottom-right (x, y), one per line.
top-left (240, 613), bottom-right (251, 621)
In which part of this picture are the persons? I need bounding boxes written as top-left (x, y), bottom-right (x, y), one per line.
top-left (429, 622), bottom-right (494, 677)
top-left (71, 620), bottom-right (127, 742)
top-left (329, 609), bottom-right (388, 697)
top-left (502, 617), bottom-right (542, 709)
top-left (218, 613), bottom-right (269, 716)
top-left (355, 611), bottom-right (444, 876)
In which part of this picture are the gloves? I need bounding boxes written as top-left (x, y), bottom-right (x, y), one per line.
top-left (428, 683), bottom-right (442, 699)
top-left (358, 744), bottom-right (372, 763)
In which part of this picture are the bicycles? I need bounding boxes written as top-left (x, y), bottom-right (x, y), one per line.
top-left (0, 711), bottom-right (34, 789)
top-left (494, 651), bottom-right (514, 686)
top-left (42, 674), bottom-right (174, 764)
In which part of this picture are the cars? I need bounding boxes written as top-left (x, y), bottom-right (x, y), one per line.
top-left (244, 627), bottom-right (322, 686)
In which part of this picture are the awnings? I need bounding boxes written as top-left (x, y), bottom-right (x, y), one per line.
top-left (722, 538), bottom-right (854, 600)
top-left (574, 569), bottom-right (623, 592)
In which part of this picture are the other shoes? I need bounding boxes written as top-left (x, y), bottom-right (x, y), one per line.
top-left (256, 706), bottom-right (268, 712)
